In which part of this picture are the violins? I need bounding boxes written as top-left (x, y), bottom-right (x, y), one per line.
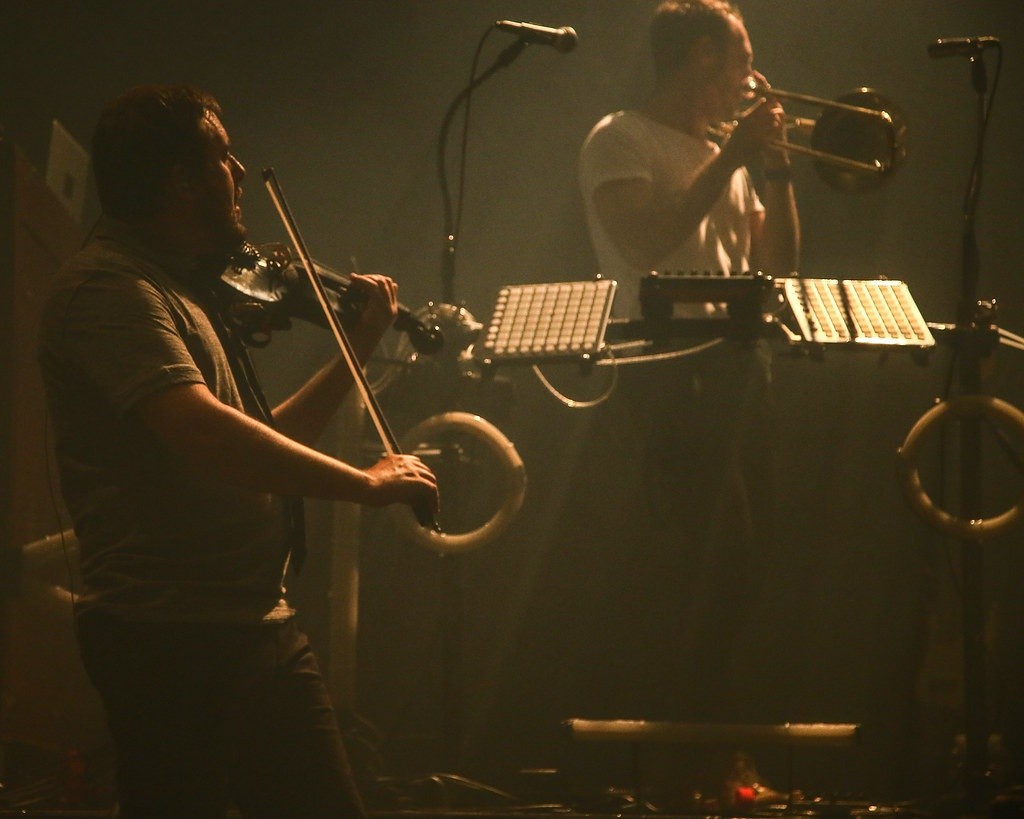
top-left (216, 240), bottom-right (446, 356)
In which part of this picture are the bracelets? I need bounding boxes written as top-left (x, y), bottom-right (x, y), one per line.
top-left (761, 165), bottom-right (793, 185)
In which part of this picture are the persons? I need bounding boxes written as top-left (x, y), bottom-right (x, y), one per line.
top-left (0, 522), bottom-right (80, 818)
top-left (557, 0), bottom-right (800, 813)
top-left (370, 301), bottom-right (519, 805)
top-left (39, 88), bottom-right (441, 819)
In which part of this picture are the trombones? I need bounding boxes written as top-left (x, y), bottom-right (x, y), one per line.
top-left (709, 82), bottom-right (911, 194)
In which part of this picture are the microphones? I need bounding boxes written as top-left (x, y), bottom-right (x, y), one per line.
top-left (495, 20), bottom-right (578, 53)
top-left (928, 36), bottom-right (999, 59)
